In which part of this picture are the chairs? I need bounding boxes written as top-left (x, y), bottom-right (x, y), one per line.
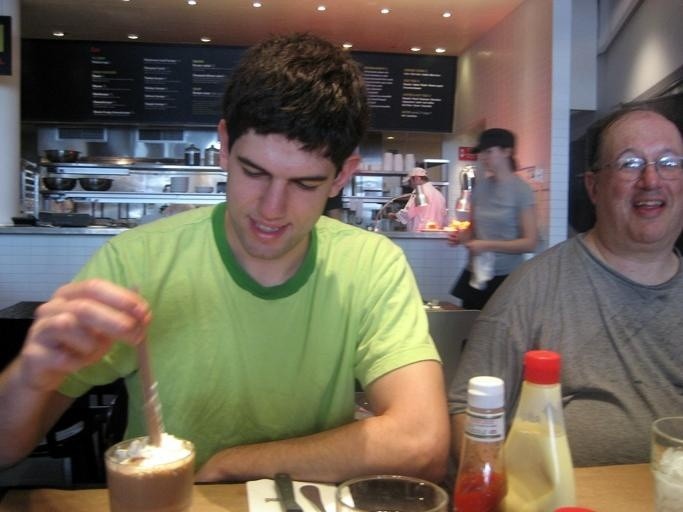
top-left (424, 305), bottom-right (482, 404)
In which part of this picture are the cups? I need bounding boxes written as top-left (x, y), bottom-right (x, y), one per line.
top-left (358, 163), bottom-right (381, 171)
top-left (382, 152), bottom-right (416, 172)
top-left (648, 416), bottom-right (683, 512)
top-left (333, 475), bottom-right (448, 512)
top-left (103, 436), bottom-right (196, 512)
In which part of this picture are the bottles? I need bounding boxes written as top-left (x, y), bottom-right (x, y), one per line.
top-left (184, 143), bottom-right (200, 166)
top-left (451, 375), bottom-right (508, 512)
top-left (500, 348), bottom-right (577, 512)
top-left (204, 144), bottom-right (220, 166)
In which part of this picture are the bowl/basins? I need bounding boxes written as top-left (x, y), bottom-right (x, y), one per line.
top-left (76, 178), bottom-right (114, 191)
top-left (40, 178), bottom-right (77, 191)
top-left (41, 149), bottom-right (80, 163)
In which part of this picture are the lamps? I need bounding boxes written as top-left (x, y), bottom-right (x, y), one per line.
top-left (369, 185), bottom-right (430, 231)
top-left (455, 165), bottom-right (480, 212)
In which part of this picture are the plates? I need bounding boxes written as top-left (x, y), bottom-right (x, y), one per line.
top-left (193, 187), bottom-right (213, 193)
top-left (170, 176), bottom-right (188, 192)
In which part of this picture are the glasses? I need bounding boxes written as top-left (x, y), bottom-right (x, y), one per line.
top-left (590, 156), bottom-right (682, 179)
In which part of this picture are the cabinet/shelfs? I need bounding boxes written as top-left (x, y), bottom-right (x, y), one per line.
top-left (29, 158), bottom-right (416, 227)
top-left (420, 156), bottom-right (451, 214)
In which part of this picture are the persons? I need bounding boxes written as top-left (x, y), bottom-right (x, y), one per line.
top-left (449, 106), bottom-right (683, 472)
top-left (387, 167), bottom-right (445, 233)
top-left (0, 30), bottom-right (450, 487)
top-left (448, 127), bottom-right (538, 308)
top-left (326, 194), bottom-right (341, 220)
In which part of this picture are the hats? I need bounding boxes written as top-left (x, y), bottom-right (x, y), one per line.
top-left (470, 128), bottom-right (514, 154)
top-left (402, 167), bottom-right (427, 183)
